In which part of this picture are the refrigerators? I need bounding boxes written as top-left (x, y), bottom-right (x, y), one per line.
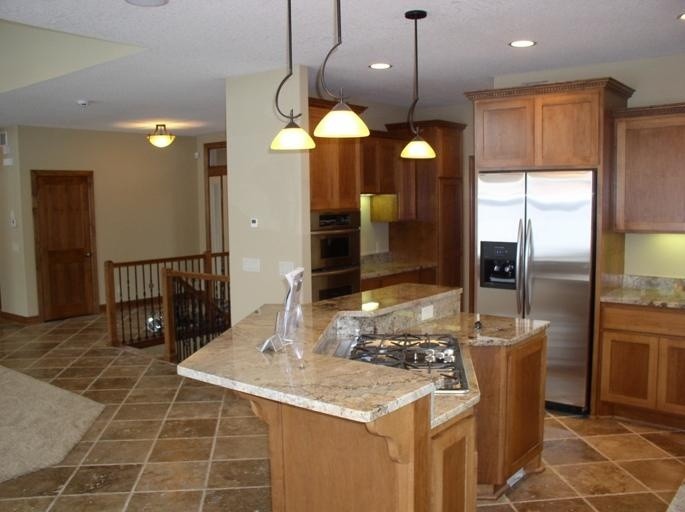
top-left (478, 171), bottom-right (594, 413)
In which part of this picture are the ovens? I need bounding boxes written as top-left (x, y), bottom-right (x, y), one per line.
top-left (311, 209), bottom-right (361, 304)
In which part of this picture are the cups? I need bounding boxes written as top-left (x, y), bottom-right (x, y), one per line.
top-left (276, 312), bottom-right (303, 345)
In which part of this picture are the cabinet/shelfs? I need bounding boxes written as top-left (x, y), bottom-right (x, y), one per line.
top-left (504, 333), bottom-right (548, 478)
top-left (598, 307), bottom-right (685, 416)
top-left (613, 112), bottom-right (685, 233)
top-left (430, 415), bottom-right (480, 512)
top-left (437, 181), bottom-right (461, 286)
top-left (474, 90), bottom-right (600, 173)
top-left (437, 127), bottom-right (462, 179)
top-left (398, 140), bottom-right (418, 222)
top-left (308, 107), bottom-right (363, 209)
top-left (361, 138), bottom-right (401, 193)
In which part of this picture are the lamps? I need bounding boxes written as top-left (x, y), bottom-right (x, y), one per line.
top-left (145, 123), bottom-right (177, 149)
top-left (266, 1), bottom-right (439, 161)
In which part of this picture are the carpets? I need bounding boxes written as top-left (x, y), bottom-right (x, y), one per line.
top-left (0, 362), bottom-right (105, 484)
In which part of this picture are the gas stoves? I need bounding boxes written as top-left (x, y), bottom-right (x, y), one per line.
top-left (344, 332), bottom-right (469, 393)
